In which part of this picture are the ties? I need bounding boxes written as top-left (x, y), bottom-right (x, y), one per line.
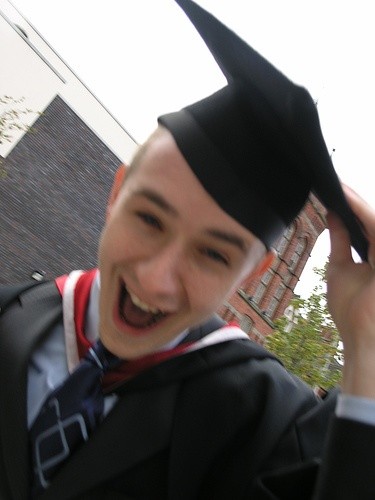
top-left (30, 340), bottom-right (126, 495)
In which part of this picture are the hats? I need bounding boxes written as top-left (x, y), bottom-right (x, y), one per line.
top-left (156, 0), bottom-right (369, 262)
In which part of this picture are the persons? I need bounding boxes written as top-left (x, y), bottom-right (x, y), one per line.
top-left (0, 0), bottom-right (374, 500)
